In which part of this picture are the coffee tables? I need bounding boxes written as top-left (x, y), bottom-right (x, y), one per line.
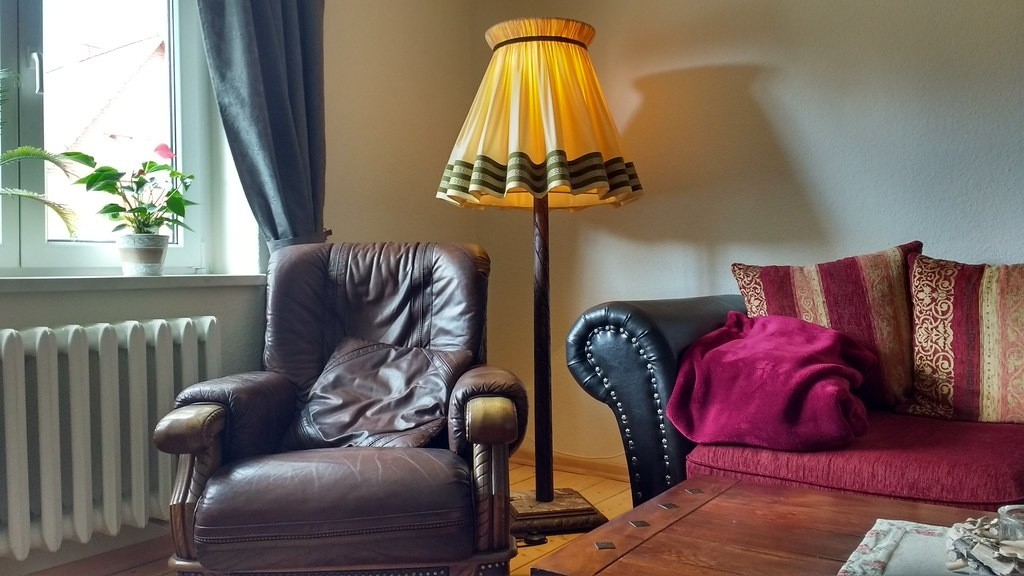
top-left (530, 475), bottom-right (998, 576)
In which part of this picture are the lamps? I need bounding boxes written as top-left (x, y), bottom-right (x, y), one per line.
top-left (433, 16), bottom-right (645, 536)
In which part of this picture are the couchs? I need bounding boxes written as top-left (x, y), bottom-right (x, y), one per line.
top-left (154, 242), bottom-right (529, 576)
top-left (565, 294), bottom-right (1024, 509)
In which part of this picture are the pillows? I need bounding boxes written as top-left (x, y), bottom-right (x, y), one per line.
top-left (895, 252), bottom-right (1024, 425)
top-left (731, 241), bottom-right (922, 412)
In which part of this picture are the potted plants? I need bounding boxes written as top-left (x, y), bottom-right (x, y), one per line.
top-left (58, 142), bottom-right (202, 277)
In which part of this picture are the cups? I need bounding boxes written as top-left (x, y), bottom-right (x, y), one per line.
top-left (998, 505), bottom-right (1024, 568)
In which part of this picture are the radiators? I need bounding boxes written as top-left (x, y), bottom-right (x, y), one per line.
top-left (0, 316), bottom-right (224, 562)
